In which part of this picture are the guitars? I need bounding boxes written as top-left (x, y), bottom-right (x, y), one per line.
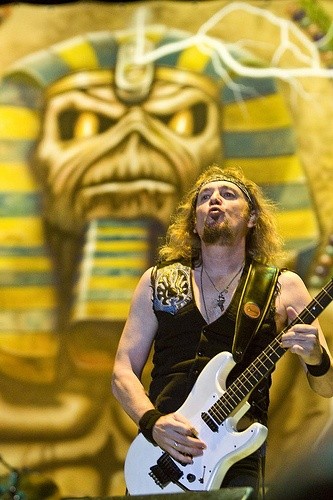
top-left (122, 277), bottom-right (332, 497)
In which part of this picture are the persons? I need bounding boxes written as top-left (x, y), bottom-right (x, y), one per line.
top-left (111, 164), bottom-right (333, 500)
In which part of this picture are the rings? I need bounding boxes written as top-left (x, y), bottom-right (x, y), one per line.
top-left (172, 442), bottom-right (178, 449)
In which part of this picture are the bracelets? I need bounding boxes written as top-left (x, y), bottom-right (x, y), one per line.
top-left (305, 345), bottom-right (331, 377)
top-left (138, 409), bottom-right (165, 447)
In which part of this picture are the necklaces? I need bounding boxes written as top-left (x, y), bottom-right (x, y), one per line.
top-left (202, 264), bottom-right (244, 312)
